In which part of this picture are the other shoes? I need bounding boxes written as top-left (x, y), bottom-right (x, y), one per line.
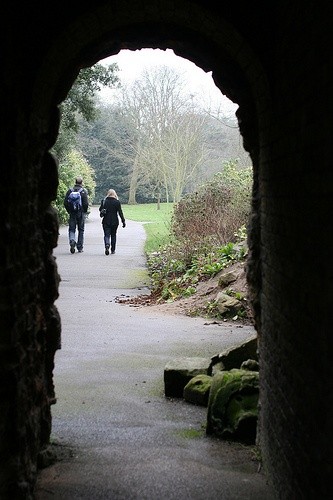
top-left (78, 250), bottom-right (83, 252)
top-left (111, 251), bottom-right (115, 254)
top-left (105, 245), bottom-right (109, 255)
top-left (70, 241), bottom-right (75, 253)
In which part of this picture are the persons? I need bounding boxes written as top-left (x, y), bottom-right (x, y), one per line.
top-left (98, 188), bottom-right (126, 255)
top-left (63, 178), bottom-right (88, 254)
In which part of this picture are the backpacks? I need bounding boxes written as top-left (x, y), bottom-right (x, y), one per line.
top-left (67, 188), bottom-right (83, 211)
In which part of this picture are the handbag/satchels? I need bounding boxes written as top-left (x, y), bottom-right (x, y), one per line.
top-left (101, 201), bottom-right (106, 217)
